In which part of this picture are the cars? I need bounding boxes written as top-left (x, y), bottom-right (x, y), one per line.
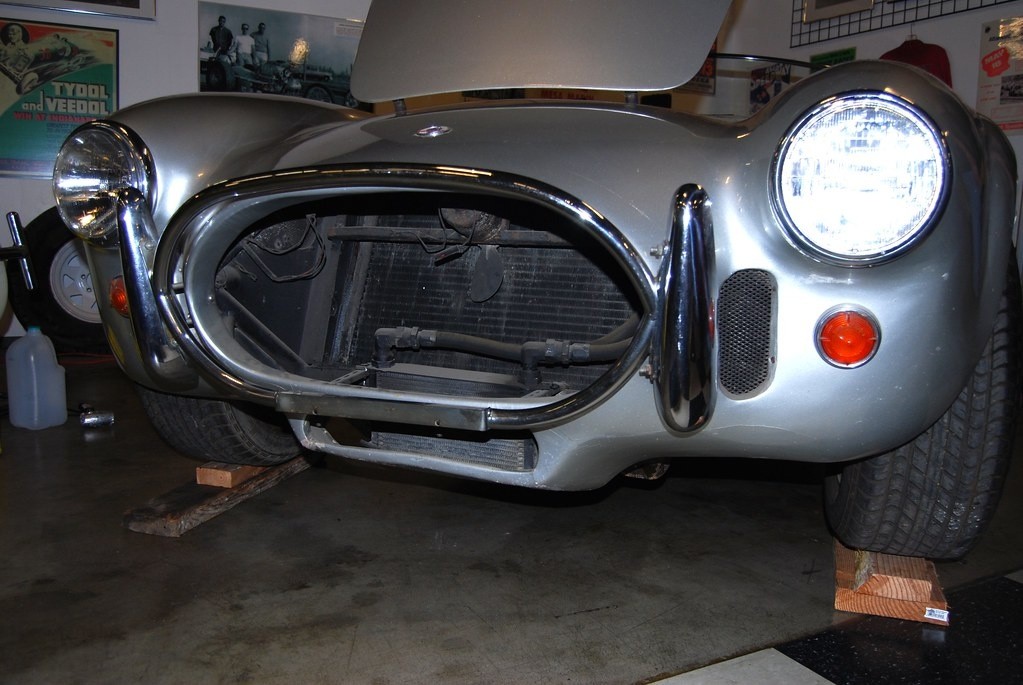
top-left (51, 0), bottom-right (1023, 562)
top-left (208, 57), bottom-right (333, 106)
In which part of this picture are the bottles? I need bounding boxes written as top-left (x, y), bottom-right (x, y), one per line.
top-left (6, 327), bottom-right (68, 431)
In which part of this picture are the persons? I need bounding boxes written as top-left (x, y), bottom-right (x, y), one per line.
top-left (229, 23), bottom-right (255, 67)
top-left (209, 16), bottom-right (233, 57)
top-left (250, 23), bottom-right (271, 64)
